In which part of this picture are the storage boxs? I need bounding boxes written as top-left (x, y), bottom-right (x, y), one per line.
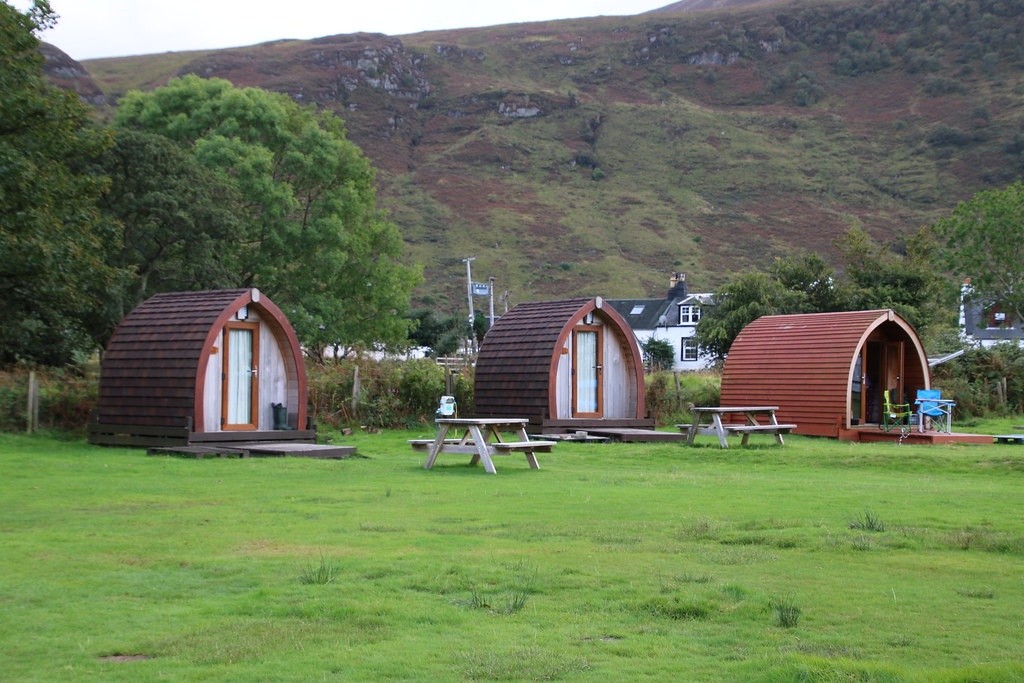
top-left (910, 414), bottom-right (918, 425)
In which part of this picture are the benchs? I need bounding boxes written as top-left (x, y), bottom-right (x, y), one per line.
top-left (675, 424), bottom-right (797, 437)
top-left (408, 439), bottom-right (558, 455)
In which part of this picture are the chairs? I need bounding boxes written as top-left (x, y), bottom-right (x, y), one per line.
top-left (914, 390), bottom-right (956, 433)
top-left (883, 390), bottom-right (912, 432)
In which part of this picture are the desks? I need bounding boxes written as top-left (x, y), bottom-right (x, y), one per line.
top-left (687, 407), bottom-right (784, 448)
top-left (425, 418), bottom-right (540, 473)
top-left (916, 399), bottom-right (955, 435)
